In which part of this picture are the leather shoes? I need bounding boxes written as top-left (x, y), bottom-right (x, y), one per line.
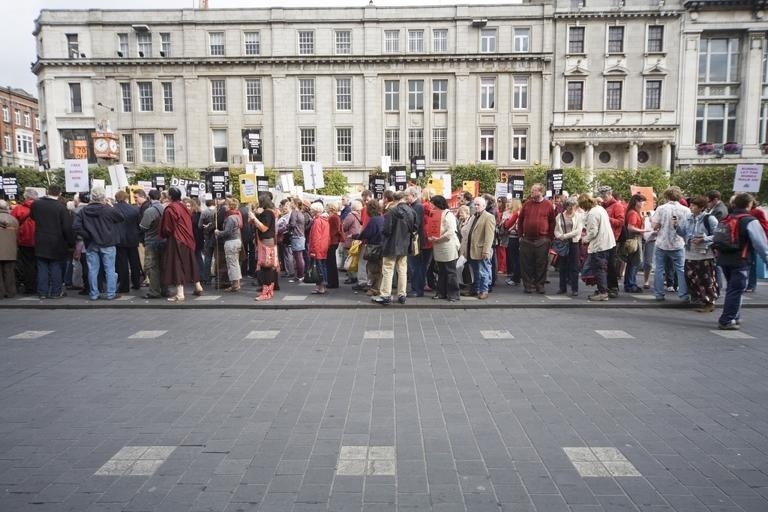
top-left (344, 279), bottom-right (356, 283)
top-left (345, 278), bottom-right (349, 281)
top-left (432, 295), bottom-right (447, 299)
top-left (557, 290), bottom-right (567, 294)
top-left (449, 297), bottom-right (455, 301)
top-left (90, 296), bottom-right (100, 301)
top-left (352, 282), bottom-right (367, 292)
top-left (143, 293), bottom-right (158, 298)
top-left (479, 293), bottom-right (489, 299)
top-left (114, 294), bottom-right (121, 298)
top-left (572, 291), bottom-right (578, 296)
top-left (463, 292), bottom-right (474, 296)
top-left (408, 290), bottom-right (424, 297)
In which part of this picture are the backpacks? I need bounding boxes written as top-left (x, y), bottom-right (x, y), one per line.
top-left (714, 210), bottom-right (751, 250)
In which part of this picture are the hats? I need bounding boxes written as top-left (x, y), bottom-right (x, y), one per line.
top-left (598, 185), bottom-right (613, 194)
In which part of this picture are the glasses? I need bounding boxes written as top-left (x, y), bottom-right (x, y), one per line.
top-left (341, 198), bottom-right (346, 200)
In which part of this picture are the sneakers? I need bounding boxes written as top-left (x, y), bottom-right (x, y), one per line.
top-left (736, 315), bottom-right (742, 323)
top-left (38, 295), bottom-right (47, 299)
top-left (625, 286), bottom-right (642, 293)
top-left (656, 298), bottom-right (664, 302)
top-left (680, 300), bottom-right (690, 302)
top-left (324, 288), bottom-right (327, 292)
top-left (697, 304), bottom-right (714, 312)
top-left (224, 286), bottom-right (240, 293)
top-left (525, 287), bottom-right (532, 293)
top-left (424, 285), bottom-right (432, 291)
top-left (588, 293), bottom-right (608, 302)
top-left (536, 286), bottom-right (544, 294)
top-left (588, 290), bottom-right (600, 298)
top-left (508, 281), bottom-right (521, 286)
top-left (398, 295), bottom-right (406, 303)
top-left (288, 279), bottom-right (295, 282)
top-left (193, 291), bottom-right (203, 296)
top-left (295, 276), bottom-right (298, 279)
top-left (609, 287), bottom-right (618, 297)
top-left (372, 295), bottom-right (391, 303)
top-left (643, 284), bottom-right (650, 289)
top-left (676, 286), bottom-right (679, 292)
top-left (168, 295), bottom-right (184, 301)
top-left (297, 277), bottom-right (304, 281)
top-left (718, 322), bottom-right (740, 330)
top-left (668, 287), bottom-right (674, 292)
top-left (79, 290), bottom-right (89, 295)
top-left (51, 291), bottom-right (67, 299)
top-left (311, 287), bottom-right (325, 293)
top-left (504, 278), bottom-right (511, 283)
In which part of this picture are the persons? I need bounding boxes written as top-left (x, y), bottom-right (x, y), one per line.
top-left (641, 190), bottom-right (658, 289)
top-left (346, 200), bottom-right (385, 296)
top-left (0, 199), bottom-right (24, 299)
top-left (481, 191), bottom-right (498, 294)
top-left (401, 186), bottom-right (428, 298)
top-left (351, 190), bottom-right (374, 292)
top-left (618, 197), bottom-right (627, 280)
top-left (651, 190), bottom-right (691, 303)
top-left (214, 197), bottom-right (250, 293)
top-left (136, 189), bottom-right (171, 299)
top-left (742, 196), bottom-right (768, 292)
top-left (342, 201), bottom-right (363, 284)
top-left (11, 186), bottom-right (41, 296)
top-left (254, 190), bottom-right (283, 292)
top-left (554, 200), bottom-right (585, 297)
top-left (426, 195), bottom-right (460, 302)
top-left (380, 189), bottom-right (395, 215)
top-left (577, 194), bottom-right (617, 302)
top-left (72, 186), bottom-right (122, 301)
top-left (282, 197), bottom-right (306, 283)
top-left (459, 198), bottom-right (496, 300)
top-left (71, 190), bottom-right (105, 294)
top-left (301, 199), bottom-right (310, 214)
top-left (672, 197), bottom-right (721, 312)
top-left (457, 192), bottom-right (477, 216)
top-left (618, 192), bottom-right (647, 293)
top-left (30, 183), bottom-right (71, 300)
top-left (516, 183), bottom-right (556, 294)
top-left (419, 189), bottom-right (439, 292)
top-left (276, 202), bottom-right (297, 280)
top-left (324, 200), bottom-right (342, 288)
top-left (130, 188), bottom-right (154, 290)
top-left (336, 195), bottom-right (355, 273)
top-left (246, 193), bottom-right (276, 301)
top-left (456, 203), bottom-right (475, 288)
top-left (701, 189), bottom-right (731, 227)
top-left (158, 189), bottom-right (170, 210)
top-left (302, 202), bottom-right (327, 295)
top-left (183, 199), bottom-right (207, 283)
top-left (212, 196), bottom-right (225, 270)
top-left (599, 184), bottom-right (620, 297)
top-left (713, 189), bottom-right (768, 333)
top-left (371, 190), bottom-right (420, 305)
top-left (199, 192), bottom-right (218, 288)
top-left (239, 200), bottom-right (258, 278)
top-left (671, 185), bottom-right (690, 207)
top-left (496, 196), bottom-right (506, 274)
top-left (110, 190), bottom-right (142, 293)
top-left (499, 198), bottom-right (521, 286)
top-left (160, 187), bottom-right (205, 302)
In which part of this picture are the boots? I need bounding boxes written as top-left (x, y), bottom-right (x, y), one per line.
top-left (255, 284), bottom-right (273, 301)
top-left (257, 282), bottom-right (275, 298)
top-left (257, 282), bottom-right (274, 296)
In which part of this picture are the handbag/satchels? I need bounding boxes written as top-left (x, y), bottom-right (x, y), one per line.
top-left (302, 252), bottom-right (326, 285)
